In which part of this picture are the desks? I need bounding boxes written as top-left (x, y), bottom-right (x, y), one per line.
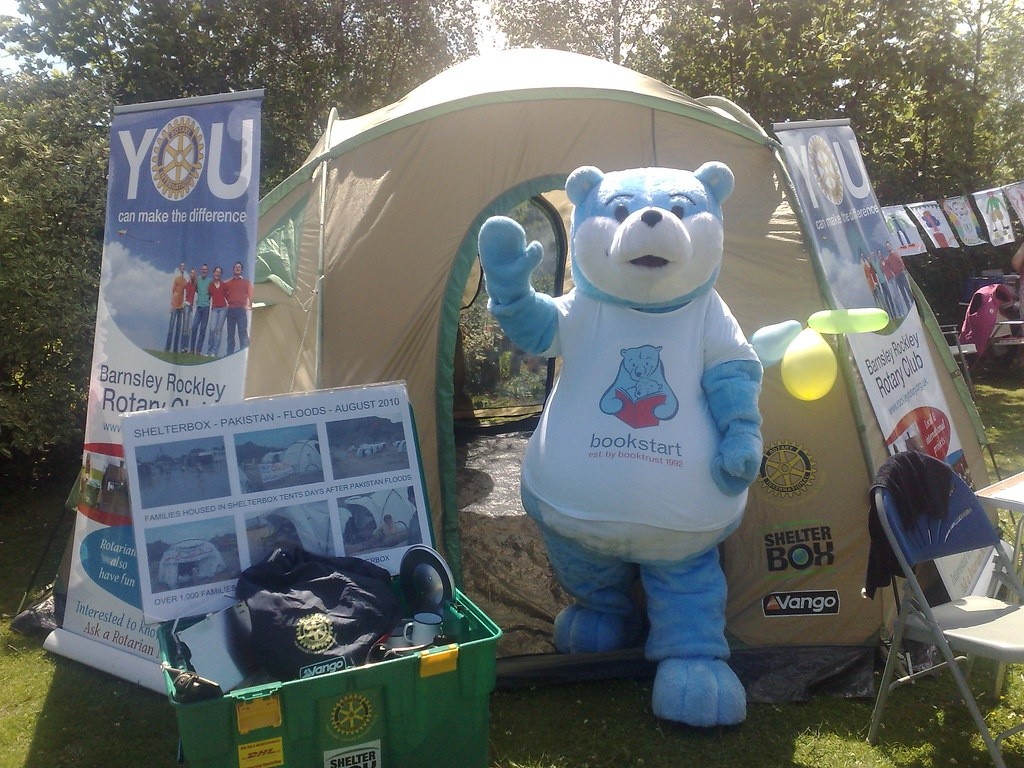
top-left (972, 470), bottom-right (1024, 702)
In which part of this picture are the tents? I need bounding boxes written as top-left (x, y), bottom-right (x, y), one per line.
top-left (262, 440), bottom-right (322, 474)
top-left (256, 501), bottom-right (335, 559)
top-left (157, 539), bottom-right (227, 588)
top-left (340, 489), bottom-right (417, 548)
top-left (357, 440), bottom-right (407, 456)
top-left (11, 53), bottom-right (1024, 700)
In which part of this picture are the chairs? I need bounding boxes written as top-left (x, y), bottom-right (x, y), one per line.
top-left (867, 452), bottom-right (1024, 768)
top-left (971, 297), bottom-right (1024, 374)
top-left (940, 325), bottom-right (979, 400)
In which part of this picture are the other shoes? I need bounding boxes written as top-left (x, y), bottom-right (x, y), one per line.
top-left (889, 314), bottom-right (905, 321)
top-left (164, 348), bottom-right (217, 359)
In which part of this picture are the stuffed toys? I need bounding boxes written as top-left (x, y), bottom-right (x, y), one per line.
top-left (478, 161), bottom-right (764, 724)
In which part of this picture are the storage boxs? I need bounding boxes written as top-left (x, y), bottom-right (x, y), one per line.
top-left (158, 405), bottom-right (504, 768)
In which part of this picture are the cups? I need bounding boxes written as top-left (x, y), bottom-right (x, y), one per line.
top-left (403, 612), bottom-right (443, 646)
top-left (386, 626), bottom-right (412, 647)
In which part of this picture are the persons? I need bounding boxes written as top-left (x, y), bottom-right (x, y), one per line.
top-left (373, 515), bottom-right (400, 540)
top-left (860, 241), bottom-right (914, 321)
top-left (345, 517), bottom-right (358, 544)
top-left (407, 487), bottom-right (423, 545)
top-left (186, 565), bottom-right (196, 582)
top-left (1011, 236), bottom-right (1024, 369)
top-left (140, 459), bottom-right (206, 489)
top-left (166, 262), bottom-right (254, 357)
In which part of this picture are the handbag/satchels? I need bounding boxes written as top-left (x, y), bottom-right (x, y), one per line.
top-left (234, 546), bottom-right (394, 682)
